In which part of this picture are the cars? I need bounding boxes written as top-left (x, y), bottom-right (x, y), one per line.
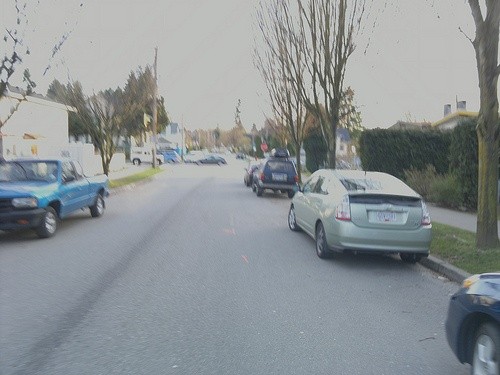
top-left (156, 149), bottom-right (178, 164)
top-left (444, 272), bottom-right (499, 375)
top-left (196, 155), bottom-right (226, 166)
top-left (287, 169), bottom-right (433, 264)
top-left (183, 150), bottom-right (203, 163)
top-left (244, 163), bottom-right (261, 186)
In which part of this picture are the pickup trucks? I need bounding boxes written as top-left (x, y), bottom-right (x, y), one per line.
top-left (0, 157), bottom-right (111, 237)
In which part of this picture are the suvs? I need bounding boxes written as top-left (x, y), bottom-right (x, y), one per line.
top-left (252, 148), bottom-right (300, 197)
top-left (130, 148), bottom-right (164, 165)
